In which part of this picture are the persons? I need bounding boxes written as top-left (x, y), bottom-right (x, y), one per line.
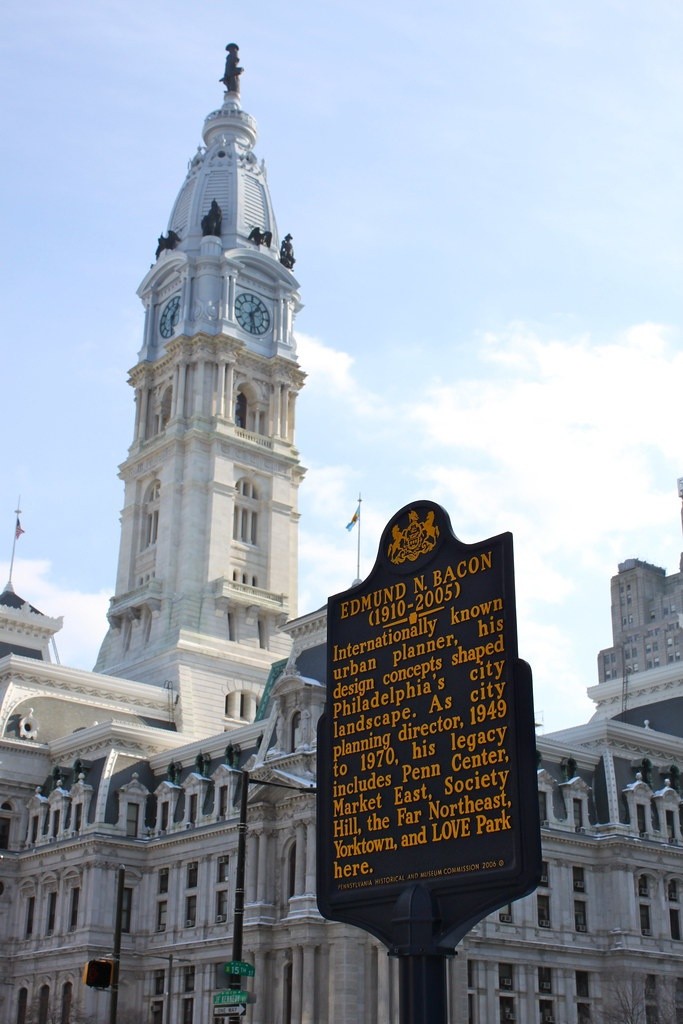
top-left (201, 200), bottom-right (221, 236)
top-left (224, 43), bottom-right (243, 93)
top-left (280, 233), bottom-right (296, 272)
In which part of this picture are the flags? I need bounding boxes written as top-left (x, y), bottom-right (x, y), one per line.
top-left (346, 506), bottom-right (360, 531)
top-left (16, 519), bottom-right (24, 539)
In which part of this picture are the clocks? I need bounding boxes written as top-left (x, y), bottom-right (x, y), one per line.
top-left (234, 293), bottom-right (270, 335)
top-left (160, 295), bottom-right (182, 339)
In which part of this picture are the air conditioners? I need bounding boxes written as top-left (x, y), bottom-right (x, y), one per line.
top-left (639, 888), bottom-right (648, 894)
top-left (185, 967), bottom-right (192, 974)
top-left (506, 1013), bottom-right (516, 1019)
top-left (28, 843), bottom-right (35, 848)
top-left (670, 892), bottom-right (679, 899)
top-left (185, 822), bottom-right (194, 829)
top-left (546, 1015), bottom-right (556, 1022)
top-left (158, 830), bottom-right (165, 836)
top-left (501, 915), bottom-right (512, 922)
top-left (216, 816), bottom-right (224, 822)
top-left (541, 876), bottom-right (547, 882)
top-left (185, 920), bottom-right (195, 927)
top-left (642, 929), bottom-right (652, 935)
top-left (160, 869), bottom-right (168, 875)
top-left (641, 833), bottom-right (649, 839)
top-left (49, 837), bottom-right (56, 842)
top-left (539, 920), bottom-right (549, 926)
top-left (540, 982), bottom-right (550, 989)
top-left (71, 831), bottom-right (79, 837)
top-left (151, 1005), bottom-right (161, 1011)
top-left (157, 924), bottom-right (165, 931)
top-left (154, 970), bottom-right (162, 977)
top-left (577, 925), bottom-right (585, 931)
top-left (577, 828), bottom-right (585, 833)
top-left (670, 838), bottom-right (678, 844)
top-left (219, 857), bottom-right (227, 863)
top-left (188, 863), bottom-right (196, 869)
top-left (216, 915), bottom-right (226, 922)
top-left (501, 978), bottom-right (512, 985)
top-left (541, 820), bottom-right (549, 827)
top-left (575, 881), bottom-right (584, 887)
